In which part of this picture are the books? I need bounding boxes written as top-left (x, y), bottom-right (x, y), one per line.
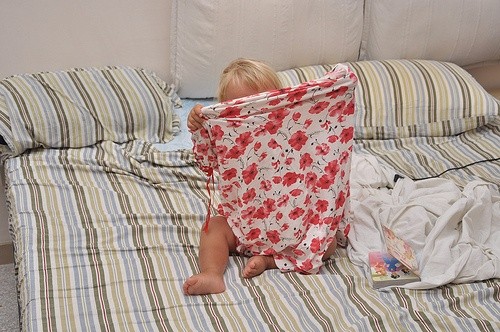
top-left (367, 223), bottom-right (422, 289)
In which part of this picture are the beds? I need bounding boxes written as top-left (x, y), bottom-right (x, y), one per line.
top-left (0, 0), bottom-right (500, 332)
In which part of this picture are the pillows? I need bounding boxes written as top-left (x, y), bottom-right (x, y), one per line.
top-left (0, 65), bottom-right (183, 157)
top-left (276, 57), bottom-right (500, 138)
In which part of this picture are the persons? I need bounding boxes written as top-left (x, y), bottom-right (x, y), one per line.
top-left (183, 58), bottom-right (359, 295)
